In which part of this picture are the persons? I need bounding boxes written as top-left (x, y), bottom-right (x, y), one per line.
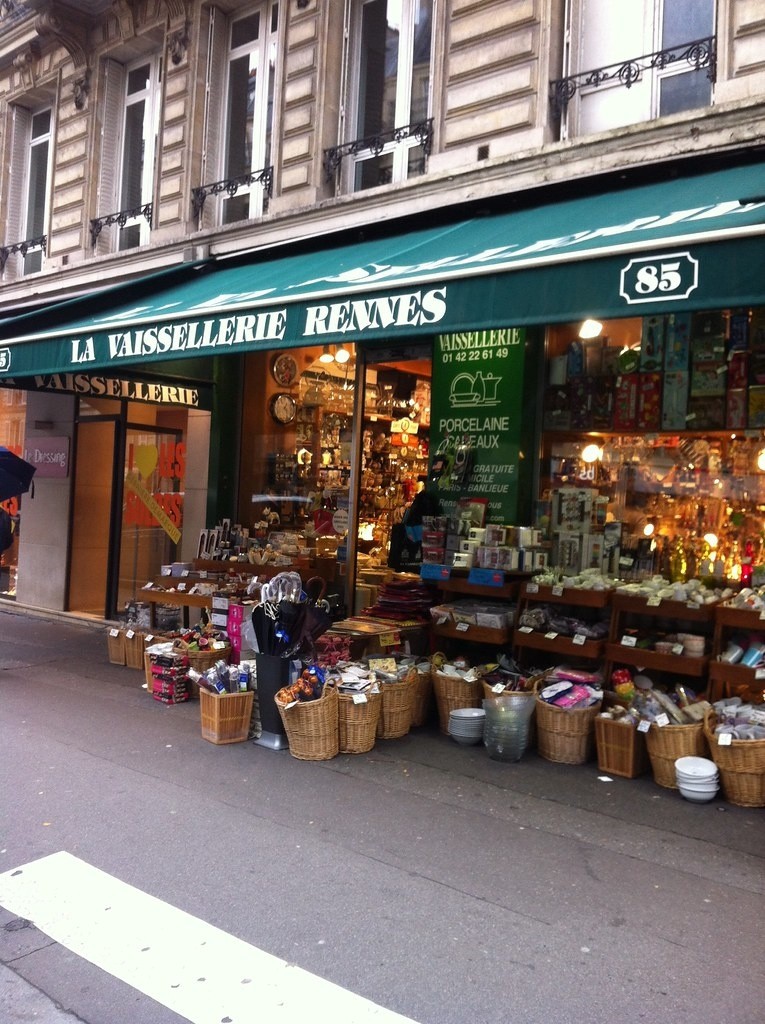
top-left (622, 483), bottom-right (651, 532)
top-left (597, 485), bottom-right (617, 521)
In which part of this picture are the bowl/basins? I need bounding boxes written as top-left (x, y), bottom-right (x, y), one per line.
top-left (446, 695), bottom-right (537, 763)
top-left (674, 755), bottom-right (721, 802)
top-left (655, 633), bottom-right (705, 658)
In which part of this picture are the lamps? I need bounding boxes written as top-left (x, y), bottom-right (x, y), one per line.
top-left (319, 344), bottom-right (334, 363)
top-left (335, 344), bottom-right (350, 363)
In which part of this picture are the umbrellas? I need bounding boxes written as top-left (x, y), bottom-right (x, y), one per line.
top-left (0, 445), bottom-right (37, 503)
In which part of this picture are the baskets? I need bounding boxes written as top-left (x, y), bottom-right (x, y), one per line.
top-left (173, 644), bottom-right (230, 698)
top-left (274, 652), bottom-right (765, 807)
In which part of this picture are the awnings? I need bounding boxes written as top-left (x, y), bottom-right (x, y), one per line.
top-left (0, 161), bottom-right (765, 410)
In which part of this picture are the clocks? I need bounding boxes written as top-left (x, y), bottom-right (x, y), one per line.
top-left (270, 392), bottom-right (297, 424)
top-left (273, 354), bottom-right (299, 388)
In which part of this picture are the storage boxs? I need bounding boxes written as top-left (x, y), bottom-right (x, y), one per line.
top-left (726, 388), bottom-right (748, 430)
top-left (661, 371), bottom-right (690, 430)
top-left (613, 374), bottom-right (638, 431)
top-left (690, 335), bottom-right (727, 363)
top-left (690, 361), bottom-right (725, 396)
top-left (637, 372), bottom-right (663, 432)
top-left (728, 350), bottom-right (748, 388)
top-left (729, 307), bottom-right (749, 351)
top-left (664, 311), bottom-right (696, 371)
top-left (747, 301), bottom-right (765, 430)
top-left (686, 397), bottom-right (725, 431)
top-left (695, 310), bottom-right (729, 338)
top-left (640, 315), bottom-right (664, 372)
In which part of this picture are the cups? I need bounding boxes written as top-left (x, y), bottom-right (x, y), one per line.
top-left (721, 644), bottom-right (762, 668)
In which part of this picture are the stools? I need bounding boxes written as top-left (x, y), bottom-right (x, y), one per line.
top-left (620, 539), bottom-right (656, 579)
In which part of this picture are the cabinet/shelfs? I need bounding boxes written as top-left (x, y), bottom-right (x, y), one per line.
top-left (428, 570), bottom-right (548, 666)
top-left (294, 402), bottom-right (765, 588)
top-left (510, 575), bottom-right (622, 685)
top-left (139, 546), bottom-right (338, 632)
top-left (604, 589), bottom-right (735, 705)
top-left (710, 597), bottom-right (765, 707)
